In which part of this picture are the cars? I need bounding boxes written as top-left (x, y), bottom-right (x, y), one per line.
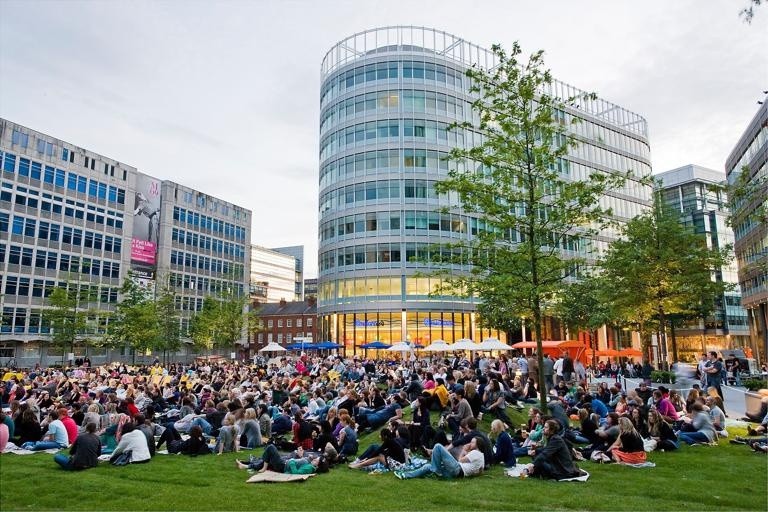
top-left (671, 362), bottom-right (697, 379)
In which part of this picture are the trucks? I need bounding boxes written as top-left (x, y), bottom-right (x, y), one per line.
top-left (724, 358), bottom-right (759, 385)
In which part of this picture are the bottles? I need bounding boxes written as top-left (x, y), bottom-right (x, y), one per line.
top-left (520, 422), bottom-right (527, 439)
top-left (209, 437), bottom-right (215, 445)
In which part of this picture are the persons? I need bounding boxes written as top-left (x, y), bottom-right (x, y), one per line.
top-left (0, 350), bottom-right (768, 480)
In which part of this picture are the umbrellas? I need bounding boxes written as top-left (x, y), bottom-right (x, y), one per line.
top-left (422, 340), bottom-right (451, 351)
top-left (471, 338), bottom-right (516, 355)
top-left (258, 341), bottom-right (287, 357)
top-left (386, 341), bottom-right (411, 351)
top-left (446, 339), bottom-right (477, 350)
